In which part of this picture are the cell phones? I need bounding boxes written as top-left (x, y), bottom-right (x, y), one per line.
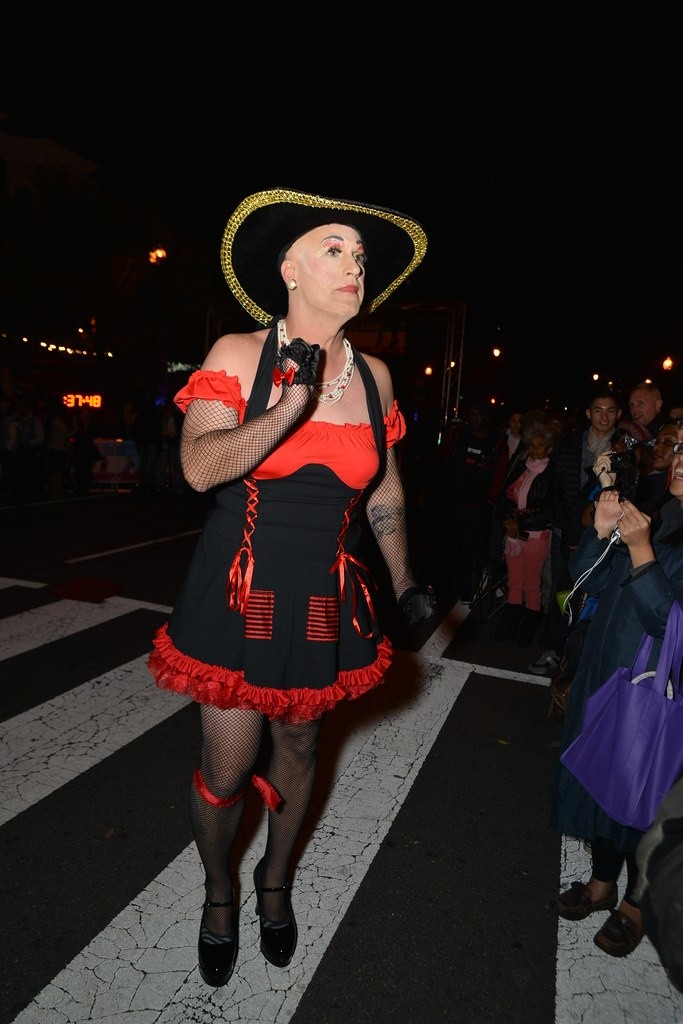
top-left (506, 528), bottom-right (530, 540)
top-left (618, 462), bottom-right (640, 506)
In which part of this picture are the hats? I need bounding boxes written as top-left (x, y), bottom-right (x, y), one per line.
top-left (219, 188), bottom-right (429, 331)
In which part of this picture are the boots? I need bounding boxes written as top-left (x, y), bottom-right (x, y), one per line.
top-left (506, 606), bottom-right (542, 653)
top-left (488, 603), bottom-right (521, 642)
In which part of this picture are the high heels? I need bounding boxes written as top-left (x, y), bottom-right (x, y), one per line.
top-left (198, 877), bottom-right (238, 988)
top-left (253, 855), bottom-right (299, 968)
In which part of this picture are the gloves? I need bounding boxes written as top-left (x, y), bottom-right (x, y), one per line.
top-left (398, 588), bottom-right (436, 626)
top-left (279, 338), bottom-right (321, 386)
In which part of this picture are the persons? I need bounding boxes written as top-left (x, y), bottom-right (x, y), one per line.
top-left (144, 189), bottom-right (435, 987)
top-left (1, 394), bottom-right (177, 510)
top-left (444, 383), bottom-right (683, 993)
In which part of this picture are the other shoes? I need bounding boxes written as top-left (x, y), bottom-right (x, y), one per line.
top-left (594, 906), bottom-right (646, 958)
top-left (528, 649), bottom-right (559, 675)
top-left (554, 878), bottom-right (620, 921)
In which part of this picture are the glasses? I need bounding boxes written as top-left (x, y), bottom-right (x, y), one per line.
top-left (673, 443), bottom-right (683, 454)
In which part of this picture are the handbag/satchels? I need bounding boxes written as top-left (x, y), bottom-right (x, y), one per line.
top-left (560, 599), bottom-right (683, 833)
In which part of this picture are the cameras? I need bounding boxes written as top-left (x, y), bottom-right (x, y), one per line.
top-left (604, 450), bottom-right (625, 473)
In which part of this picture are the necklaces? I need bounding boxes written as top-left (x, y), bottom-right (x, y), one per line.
top-left (277, 319), bottom-right (355, 406)
